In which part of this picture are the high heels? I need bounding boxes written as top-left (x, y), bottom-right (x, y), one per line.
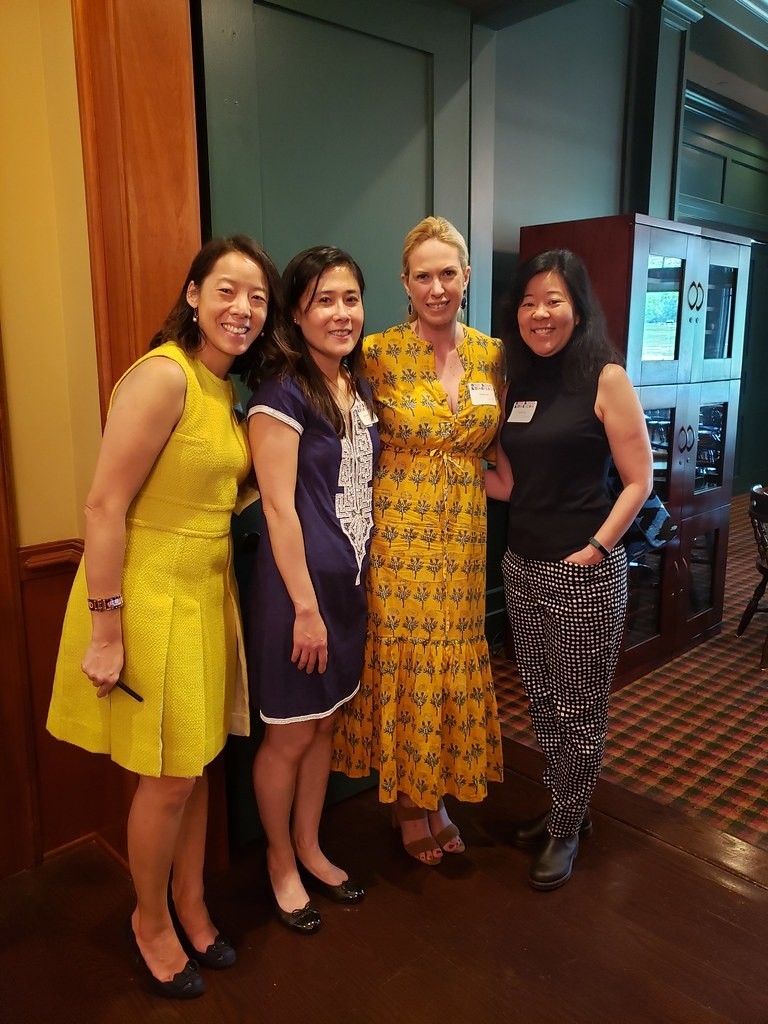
top-left (391, 802), bottom-right (441, 866)
top-left (296, 856), bottom-right (365, 904)
top-left (264, 857), bottom-right (322, 936)
top-left (169, 899), bottom-right (236, 969)
top-left (428, 799), bottom-right (465, 854)
top-left (128, 913), bottom-right (205, 997)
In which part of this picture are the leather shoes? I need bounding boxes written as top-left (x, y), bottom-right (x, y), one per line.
top-left (529, 832), bottom-right (579, 890)
top-left (511, 807), bottom-right (592, 848)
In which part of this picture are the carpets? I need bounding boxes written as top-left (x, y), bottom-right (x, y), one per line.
top-left (480, 485), bottom-right (768, 854)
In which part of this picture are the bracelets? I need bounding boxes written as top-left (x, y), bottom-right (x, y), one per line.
top-left (87, 594), bottom-right (123, 611)
top-left (588, 537), bottom-right (610, 558)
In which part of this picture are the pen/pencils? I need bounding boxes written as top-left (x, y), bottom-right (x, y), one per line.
top-left (116, 680), bottom-right (143, 702)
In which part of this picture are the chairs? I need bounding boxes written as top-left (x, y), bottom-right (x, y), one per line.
top-left (736, 484), bottom-right (768, 669)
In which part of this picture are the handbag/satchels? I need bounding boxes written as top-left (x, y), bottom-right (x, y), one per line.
top-left (609, 465), bottom-right (677, 562)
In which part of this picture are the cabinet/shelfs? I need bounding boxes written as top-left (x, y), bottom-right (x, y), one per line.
top-left (511, 213), bottom-right (752, 697)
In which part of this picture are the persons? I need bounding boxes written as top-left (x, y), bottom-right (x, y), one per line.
top-left (46, 236), bottom-right (252, 1000)
top-left (484, 249), bottom-right (654, 892)
top-left (243, 245), bottom-right (381, 934)
top-left (355, 217), bottom-right (505, 865)
